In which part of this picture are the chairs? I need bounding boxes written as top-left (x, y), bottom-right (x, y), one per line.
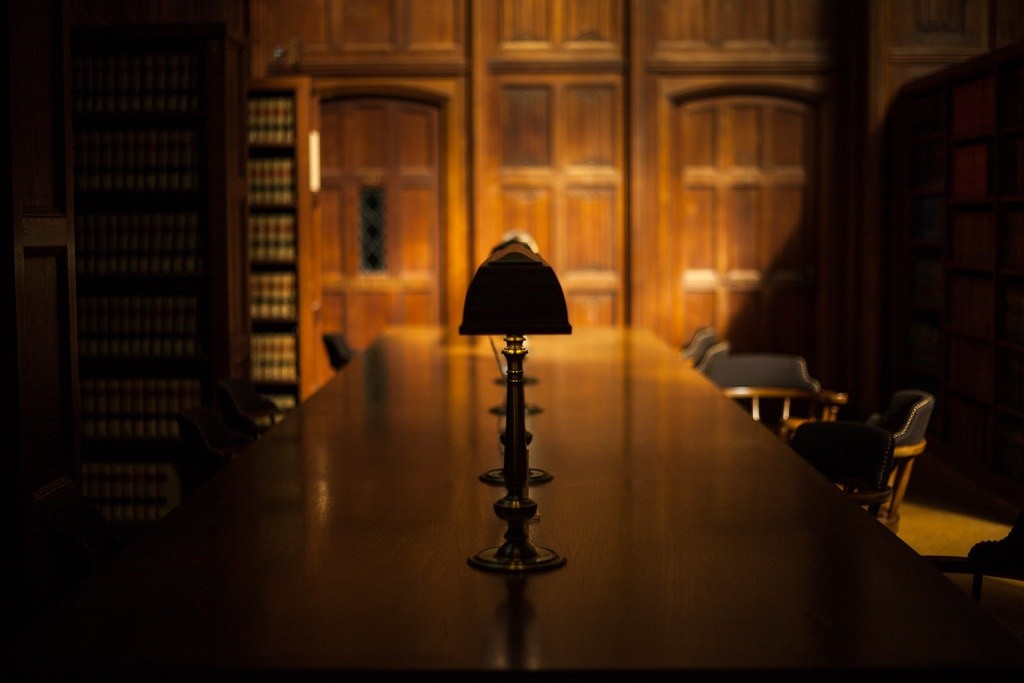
top-left (678, 327), bottom-right (1023, 601)
top-left (22, 330), bottom-right (358, 598)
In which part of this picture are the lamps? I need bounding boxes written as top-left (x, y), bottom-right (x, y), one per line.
top-left (456, 231), bottom-right (568, 574)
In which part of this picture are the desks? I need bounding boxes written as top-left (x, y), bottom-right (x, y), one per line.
top-left (0, 326), bottom-right (1023, 683)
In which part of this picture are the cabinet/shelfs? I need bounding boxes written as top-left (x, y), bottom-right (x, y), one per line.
top-left (0, 0), bottom-right (248, 542)
top-left (241, 74), bottom-right (314, 440)
top-left (882, 36), bottom-right (1023, 508)
